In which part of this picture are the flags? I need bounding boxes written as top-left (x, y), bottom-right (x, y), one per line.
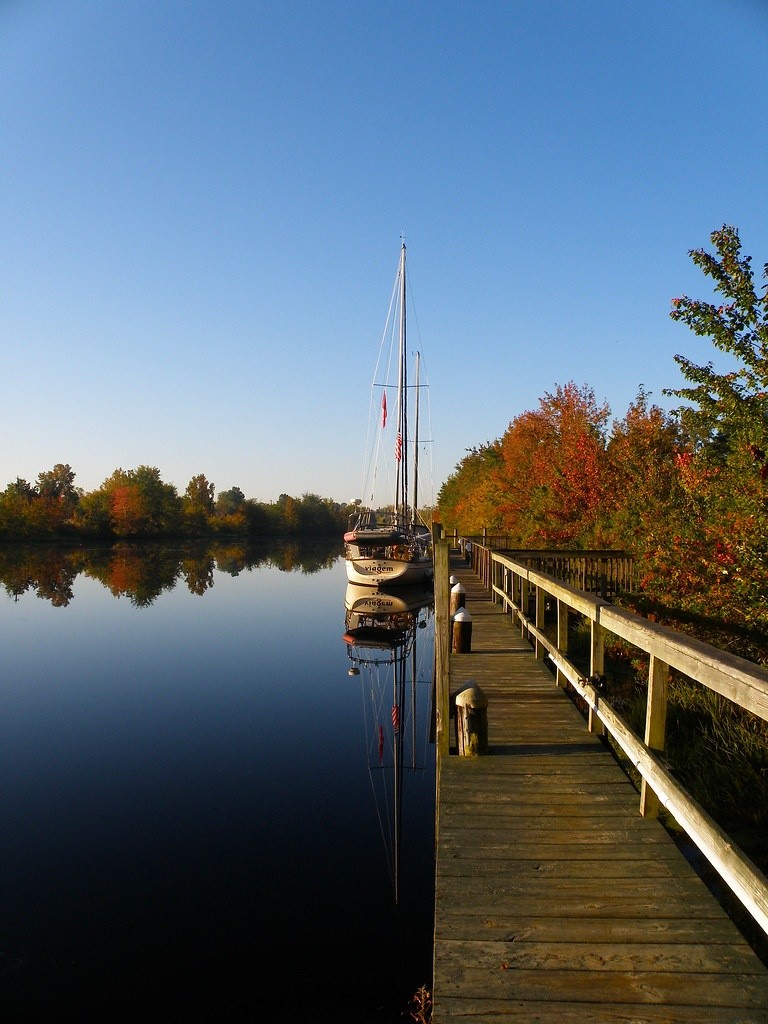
top-left (381, 393), bottom-right (387, 428)
top-left (396, 432), bottom-right (402, 462)
top-left (391, 705), bottom-right (398, 727)
top-left (378, 726), bottom-right (383, 762)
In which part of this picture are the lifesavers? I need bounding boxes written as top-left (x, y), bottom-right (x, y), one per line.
top-left (393, 545), bottom-right (409, 560)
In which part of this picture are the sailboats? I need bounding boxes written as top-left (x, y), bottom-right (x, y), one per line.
top-left (340, 582), bottom-right (426, 908)
top-left (341, 230), bottom-right (437, 593)
